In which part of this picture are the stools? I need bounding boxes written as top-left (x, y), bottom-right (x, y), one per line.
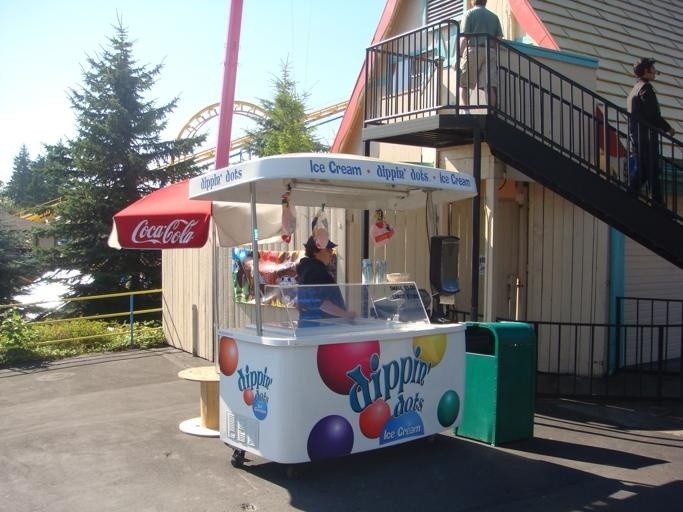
top-left (178, 366), bottom-right (220, 437)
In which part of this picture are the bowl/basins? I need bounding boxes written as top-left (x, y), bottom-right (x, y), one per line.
top-left (386, 273), bottom-right (401, 283)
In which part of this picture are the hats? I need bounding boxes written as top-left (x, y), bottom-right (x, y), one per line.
top-left (306, 236), bottom-right (337, 250)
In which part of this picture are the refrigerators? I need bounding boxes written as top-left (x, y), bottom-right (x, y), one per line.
top-left (218, 282), bottom-right (466, 475)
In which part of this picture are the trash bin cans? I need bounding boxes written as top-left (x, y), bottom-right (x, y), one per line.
top-left (455, 321), bottom-right (537, 444)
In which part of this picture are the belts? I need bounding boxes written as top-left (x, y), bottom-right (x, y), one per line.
top-left (467, 43), bottom-right (496, 50)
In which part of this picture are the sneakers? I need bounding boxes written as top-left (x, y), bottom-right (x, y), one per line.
top-left (652, 201), bottom-right (669, 213)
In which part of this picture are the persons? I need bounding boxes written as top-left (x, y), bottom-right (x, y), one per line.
top-left (626, 57), bottom-right (675, 204)
top-left (460, 0), bottom-right (503, 114)
top-left (296, 236), bottom-right (357, 328)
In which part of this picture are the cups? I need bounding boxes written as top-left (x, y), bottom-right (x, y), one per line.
top-left (362, 259), bottom-right (374, 284)
top-left (374, 259), bottom-right (386, 283)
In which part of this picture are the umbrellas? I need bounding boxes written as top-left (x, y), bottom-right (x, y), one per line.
top-left (107, 178), bottom-right (296, 336)
